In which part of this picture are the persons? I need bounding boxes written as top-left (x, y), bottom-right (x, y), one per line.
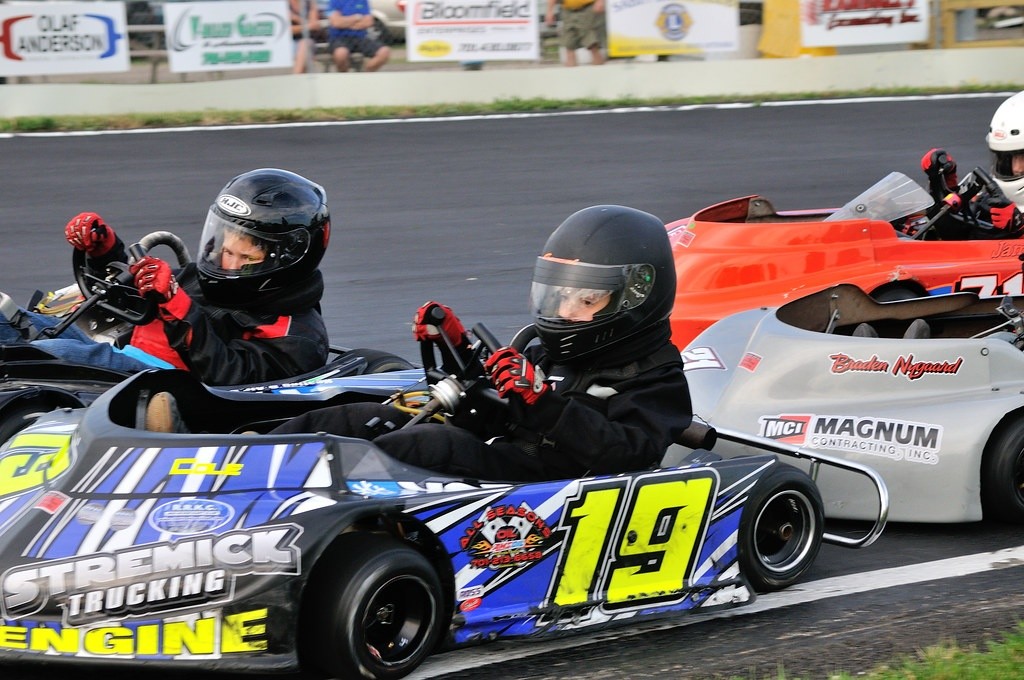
top-left (915, 90), bottom-right (1024, 240)
top-left (64, 168), bottom-right (333, 387)
top-left (147, 205), bottom-right (694, 483)
top-left (853, 252), bottom-right (1024, 343)
top-left (287, 0), bottom-right (391, 74)
top-left (545, 2), bottom-right (606, 69)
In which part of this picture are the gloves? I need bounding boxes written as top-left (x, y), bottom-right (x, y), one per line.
top-left (412, 301), bottom-right (465, 346)
top-left (921, 148), bottom-right (957, 188)
top-left (130, 256), bottom-right (192, 321)
top-left (987, 198), bottom-right (1024, 234)
top-left (64, 212), bottom-right (116, 256)
top-left (486, 346), bottom-right (548, 405)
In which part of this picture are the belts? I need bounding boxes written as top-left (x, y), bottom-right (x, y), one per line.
top-left (568, 1), bottom-right (596, 14)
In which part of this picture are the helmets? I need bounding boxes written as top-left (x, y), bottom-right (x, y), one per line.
top-left (986, 90), bottom-right (1024, 213)
top-left (535, 204), bottom-right (676, 360)
top-left (197, 168), bottom-right (331, 299)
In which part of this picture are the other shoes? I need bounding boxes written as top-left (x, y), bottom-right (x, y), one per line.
top-left (852, 323), bottom-right (879, 338)
top-left (903, 318), bottom-right (930, 338)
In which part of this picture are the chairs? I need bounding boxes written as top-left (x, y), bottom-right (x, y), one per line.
top-left (314, 30), bottom-right (363, 73)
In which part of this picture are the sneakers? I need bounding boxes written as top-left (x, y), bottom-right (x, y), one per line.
top-left (145, 392), bottom-right (190, 434)
